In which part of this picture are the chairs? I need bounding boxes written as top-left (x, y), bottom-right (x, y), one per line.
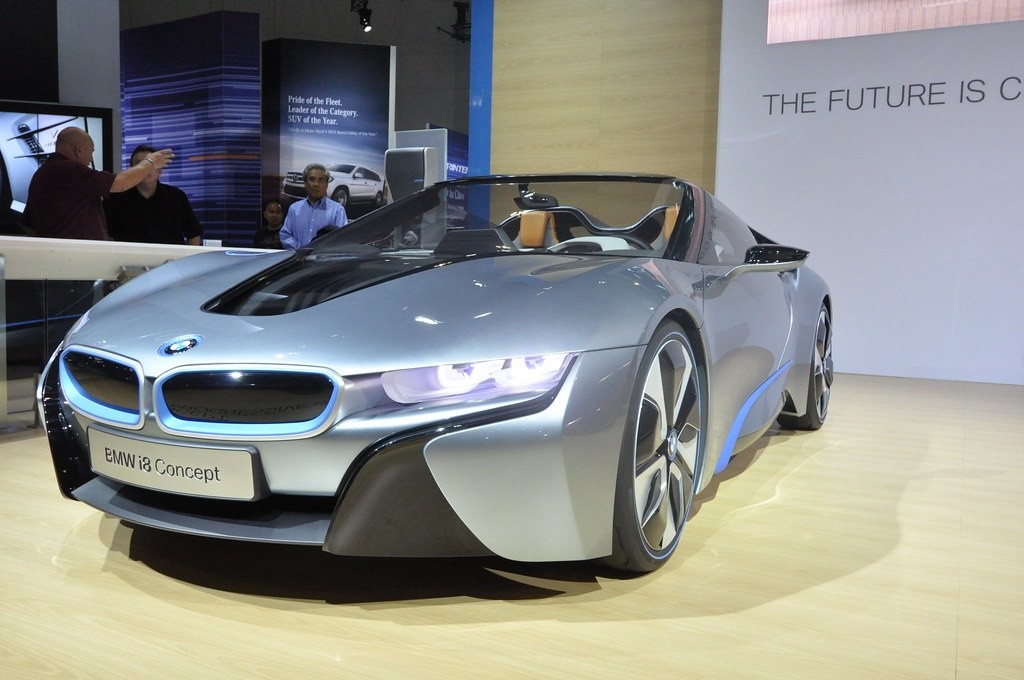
top-left (432, 228), bottom-right (518, 255)
top-left (651, 206), bottom-right (681, 250)
top-left (512, 193), bottom-right (560, 249)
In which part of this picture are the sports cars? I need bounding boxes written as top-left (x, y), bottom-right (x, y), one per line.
top-left (37, 171), bottom-right (832, 580)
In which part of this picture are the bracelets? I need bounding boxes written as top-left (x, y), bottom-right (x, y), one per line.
top-left (146, 158), bottom-right (156, 169)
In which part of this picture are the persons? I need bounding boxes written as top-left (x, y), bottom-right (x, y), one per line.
top-left (102, 145), bottom-right (203, 290)
top-left (252, 202), bottom-right (284, 249)
top-left (21, 127), bottom-right (175, 374)
top-left (0, 151), bottom-right (13, 223)
top-left (280, 164), bottom-right (348, 248)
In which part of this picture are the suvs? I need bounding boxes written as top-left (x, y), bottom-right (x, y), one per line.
top-left (281, 162), bottom-right (384, 207)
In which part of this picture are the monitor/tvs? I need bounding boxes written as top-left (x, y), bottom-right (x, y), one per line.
top-left (0, 100), bottom-right (112, 220)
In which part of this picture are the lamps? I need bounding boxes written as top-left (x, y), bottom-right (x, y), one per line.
top-left (351, 0), bottom-right (372, 32)
